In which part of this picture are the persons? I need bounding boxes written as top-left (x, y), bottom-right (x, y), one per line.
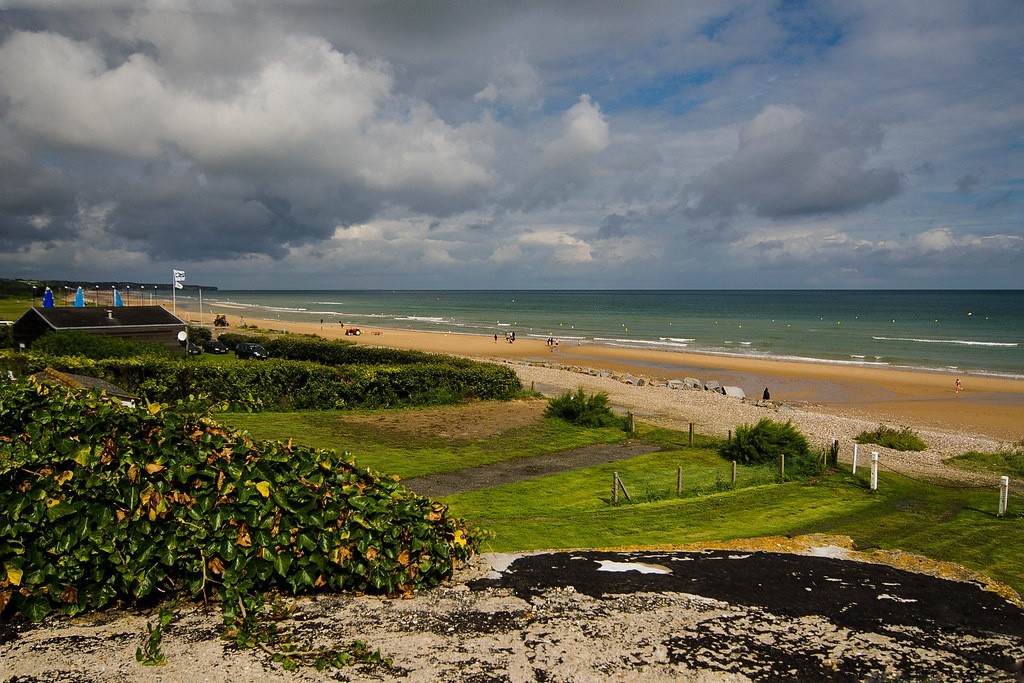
top-left (494, 330), bottom-right (516, 343)
top-left (955, 378), bottom-right (964, 394)
top-left (762, 387), bottom-right (770, 399)
top-left (546, 337), bottom-right (559, 346)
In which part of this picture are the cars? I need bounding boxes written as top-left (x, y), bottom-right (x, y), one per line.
top-left (202, 340), bottom-right (229, 354)
top-left (235, 343), bottom-right (269, 360)
top-left (188, 341), bottom-right (201, 355)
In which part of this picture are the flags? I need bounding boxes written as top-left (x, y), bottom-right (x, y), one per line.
top-left (175, 270), bottom-right (186, 289)
top-left (115, 290), bottom-right (123, 306)
